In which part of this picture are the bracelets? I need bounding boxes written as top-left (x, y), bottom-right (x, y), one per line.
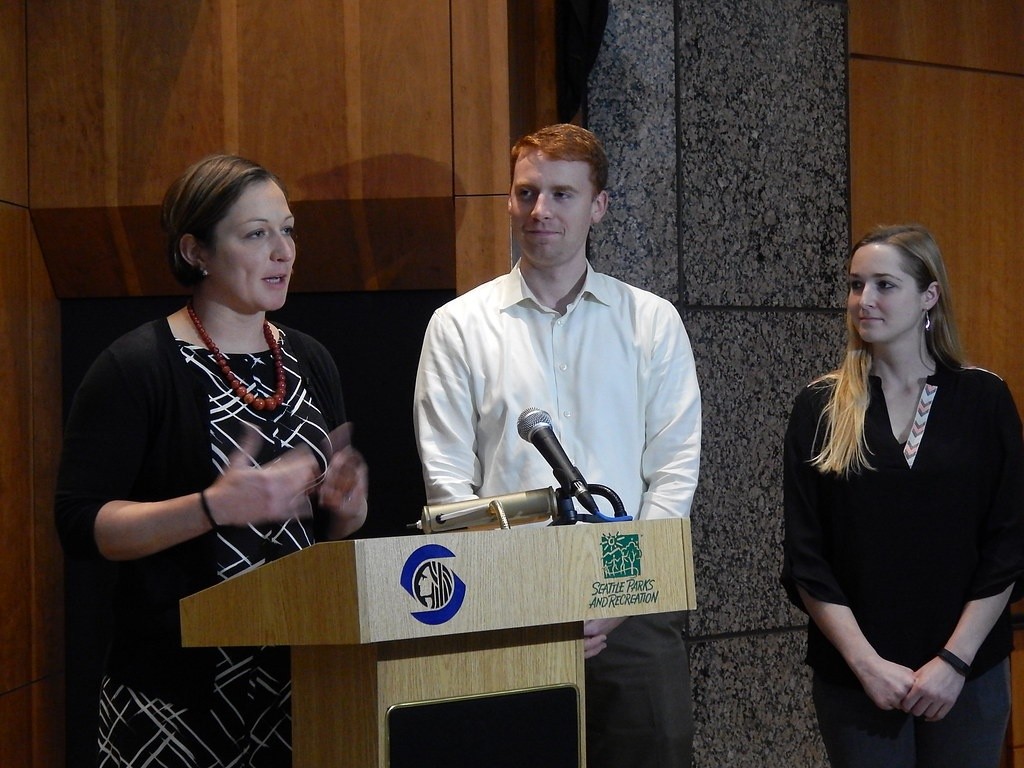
top-left (938, 648), bottom-right (970, 678)
top-left (197, 490), bottom-right (220, 529)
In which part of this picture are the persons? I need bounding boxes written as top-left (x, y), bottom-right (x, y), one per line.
top-left (48, 153), bottom-right (375, 766)
top-left (779, 217), bottom-right (1024, 766)
top-left (410, 124), bottom-right (701, 766)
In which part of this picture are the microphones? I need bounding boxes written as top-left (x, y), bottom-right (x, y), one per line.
top-left (517, 408), bottom-right (601, 512)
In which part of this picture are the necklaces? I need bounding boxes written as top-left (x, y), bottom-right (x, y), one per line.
top-left (185, 302), bottom-right (288, 412)
